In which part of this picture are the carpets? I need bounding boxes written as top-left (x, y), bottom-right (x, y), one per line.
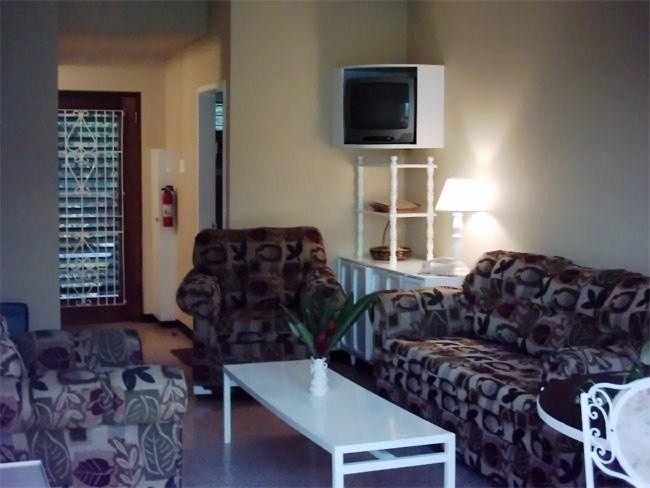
top-left (179, 433), bottom-right (496, 488)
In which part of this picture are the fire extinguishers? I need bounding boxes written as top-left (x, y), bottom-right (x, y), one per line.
top-left (162, 185), bottom-right (177, 227)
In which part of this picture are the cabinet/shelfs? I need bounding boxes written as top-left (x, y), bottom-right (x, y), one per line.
top-left (335, 155), bottom-right (466, 366)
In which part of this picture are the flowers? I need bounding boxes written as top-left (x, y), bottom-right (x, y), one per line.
top-left (275, 284), bottom-right (380, 359)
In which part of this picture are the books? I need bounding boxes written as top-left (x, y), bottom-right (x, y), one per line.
top-left (367, 199), bottom-right (421, 213)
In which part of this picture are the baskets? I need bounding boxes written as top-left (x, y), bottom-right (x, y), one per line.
top-left (368, 244), bottom-right (413, 260)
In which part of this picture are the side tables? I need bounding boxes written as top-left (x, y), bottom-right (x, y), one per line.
top-left (536, 370), bottom-right (650, 452)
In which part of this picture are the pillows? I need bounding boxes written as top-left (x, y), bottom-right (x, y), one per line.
top-left (461, 295), bottom-right (599, 360)
top-left (242, 275), bottom-right (286, 312)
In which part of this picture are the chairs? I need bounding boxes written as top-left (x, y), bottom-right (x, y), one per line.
top-left (580, 376), bottom-right (650, 488)
top-left (175, 226), bottom-right (346, 399)
top-left (0, 313), bottom-right (189, 488)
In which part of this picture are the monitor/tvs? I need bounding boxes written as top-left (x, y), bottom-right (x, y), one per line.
top-left (344, 70), bottom-right (415, 144)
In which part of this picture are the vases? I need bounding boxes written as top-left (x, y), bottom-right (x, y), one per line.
top-left (309, 356), bottom-right (332, 397)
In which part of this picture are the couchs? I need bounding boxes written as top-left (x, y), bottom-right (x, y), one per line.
top-left (365, 250), bottom-right (650, 488)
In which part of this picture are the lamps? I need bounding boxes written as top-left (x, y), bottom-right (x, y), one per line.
top-left (435, 178), bottom-right (490, 272)
